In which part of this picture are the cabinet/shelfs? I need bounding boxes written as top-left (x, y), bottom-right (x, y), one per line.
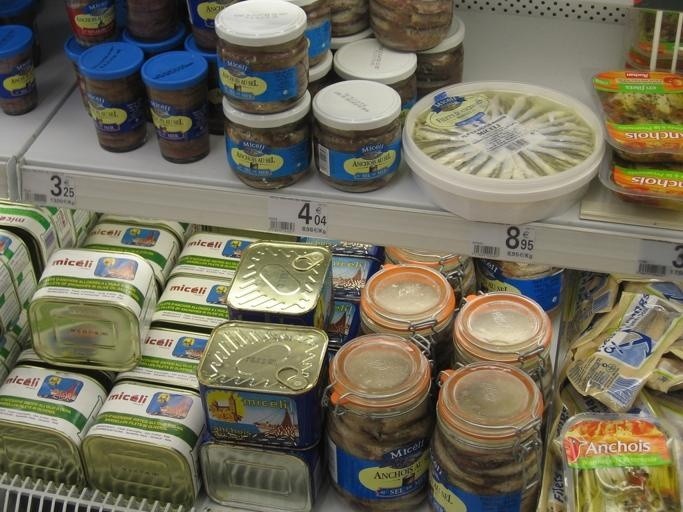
top-left (0, 0), bottom-right (683, 512)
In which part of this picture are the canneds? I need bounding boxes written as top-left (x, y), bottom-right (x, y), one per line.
top-left (0, 0), bottom-right (465, 211)
top-left (329, 248), bottom-right (565, 511)
top-left (0, 198), bottom-right (385, 512)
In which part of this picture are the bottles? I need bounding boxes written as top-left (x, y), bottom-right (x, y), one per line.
top-left (0, 0), bottom-right (241, 164)
